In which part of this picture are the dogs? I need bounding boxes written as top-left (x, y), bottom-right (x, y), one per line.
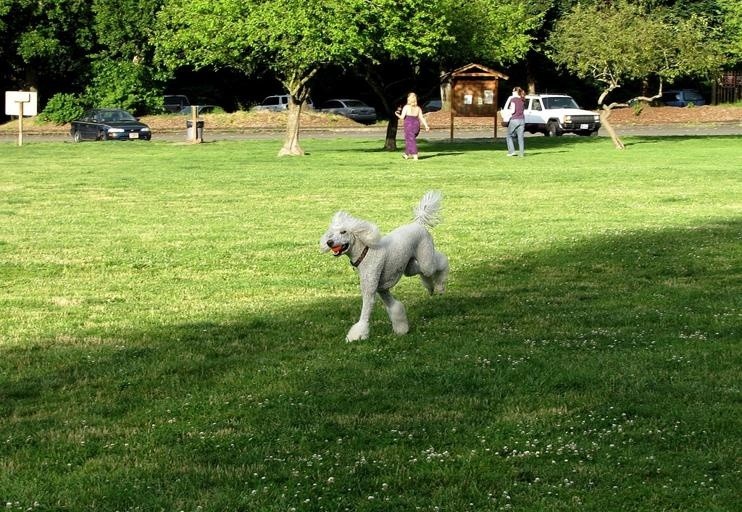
top-left (320, 189), bottom-right (449, 343)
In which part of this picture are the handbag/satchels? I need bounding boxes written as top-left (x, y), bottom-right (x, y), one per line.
top-left (501, 121), bottom-right (510, 128)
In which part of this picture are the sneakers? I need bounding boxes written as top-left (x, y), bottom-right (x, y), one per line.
top-left (506, 152), bottom-right (517, 157)
top-left (400, 153), bottom-right (409, 160)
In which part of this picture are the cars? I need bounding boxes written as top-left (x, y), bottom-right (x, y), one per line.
top-left (69, 109), bottom-right (152, 142)
top-left (178, 105), bottom-right (224, 115)
top-left (422, 99), bottom-right (442, 111)
top-left (320, 99), bottom-right (377, 123)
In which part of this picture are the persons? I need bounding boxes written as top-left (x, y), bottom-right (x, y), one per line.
top-left (506, 86), bottom-right (527, 157)
top-left (394, 92), bottom-right (430, 160)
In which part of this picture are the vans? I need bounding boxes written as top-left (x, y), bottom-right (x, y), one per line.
top-left (158, 93), bottom-right (190, 114)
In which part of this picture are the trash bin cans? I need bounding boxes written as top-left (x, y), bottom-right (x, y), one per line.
top-left (186, 119), bottom-right (204, 142)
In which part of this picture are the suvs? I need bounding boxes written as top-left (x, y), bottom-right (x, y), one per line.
top-left (501, 92), bottom-right (600, 138)
top-left (251, 94), bottom-right (316, 112)
top-left (654, 89), bottom-right (706, 107)
